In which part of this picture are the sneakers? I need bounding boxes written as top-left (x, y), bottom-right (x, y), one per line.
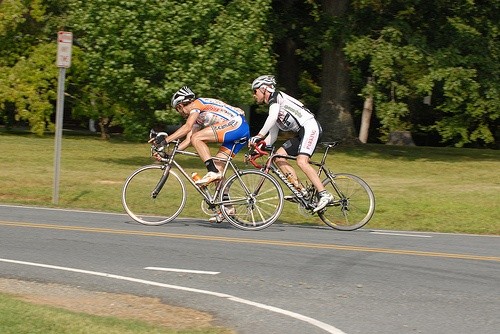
top-left (208, 207), bottom-right (236, 223)
top-left (313, 193), bottom-right (334, 213)
top-left (284, 188), bottom-right (307, 200)
top-left (195, 171), bottom-right (223, 186)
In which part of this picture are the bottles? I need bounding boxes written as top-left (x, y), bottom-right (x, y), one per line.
top-left (285, 172), bottom-right (303, 190)
top-left (190, 172), bottom-right (203, 187)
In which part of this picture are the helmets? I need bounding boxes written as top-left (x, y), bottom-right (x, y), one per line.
top-left (251, 74), bottom-right (277, 90)
top-left (171, 85), bottom-right (195, 108)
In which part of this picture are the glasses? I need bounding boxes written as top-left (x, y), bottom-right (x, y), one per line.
top-left (253, 87), bottom-right (261, 94)
top-left (175, 105), bottom-right (179, 112)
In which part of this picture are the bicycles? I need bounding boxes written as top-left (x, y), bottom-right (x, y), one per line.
top-left (122, 129), bottom-right (284, 231)
top-left (225, 140), bottom-right (376, 231)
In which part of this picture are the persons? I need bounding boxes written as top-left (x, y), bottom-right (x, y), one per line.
top-left (243, 74), bottom-right (334, 213)
top-left (153, 86), bottom-right (250, 223)
